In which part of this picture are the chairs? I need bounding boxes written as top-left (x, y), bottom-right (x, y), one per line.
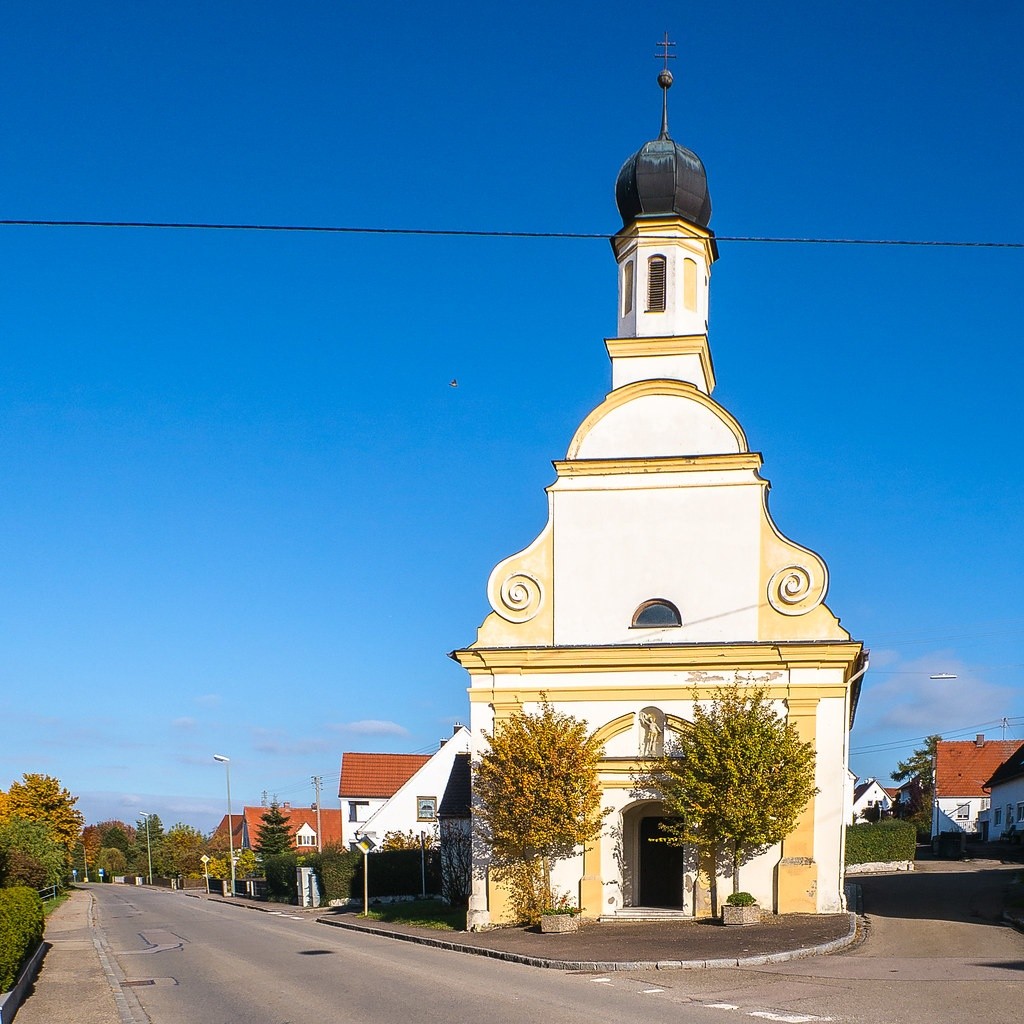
top-left (1000, 825), bottom-right (1016, 840)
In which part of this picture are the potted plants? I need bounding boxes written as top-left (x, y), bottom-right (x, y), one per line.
top-left (721, 891), bottom-right (760, 926)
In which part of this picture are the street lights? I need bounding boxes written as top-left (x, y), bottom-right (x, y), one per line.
top-left (77, 842), bottom-right (87, 882)
top-left (139, 812), bottom-right (152, 885)
top-left (214, 754), bottom-right (236, 898)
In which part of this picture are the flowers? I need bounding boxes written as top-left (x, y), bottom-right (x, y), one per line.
top-left (536, 890), bottom-right (586, 916)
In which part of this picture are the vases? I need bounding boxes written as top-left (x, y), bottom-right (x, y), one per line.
top-left (540, 913), bottom-right (581, 934)
